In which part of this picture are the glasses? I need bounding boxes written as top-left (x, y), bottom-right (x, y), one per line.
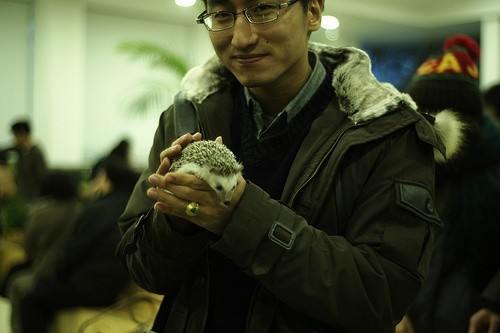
top-left (196, 0), bottom-right (294, 32)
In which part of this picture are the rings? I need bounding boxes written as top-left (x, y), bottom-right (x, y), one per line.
top-left (184, 201), bottom-right (200, 217)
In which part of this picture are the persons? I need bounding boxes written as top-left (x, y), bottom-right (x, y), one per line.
top-left (114, 0), bottom-right (446, 333)
top-left (382, 31), bottom-right (500, 332)
top-left (467, 271), bottom-right (500, 333)
top-left (1, 103), bottom-right (142, 333)
